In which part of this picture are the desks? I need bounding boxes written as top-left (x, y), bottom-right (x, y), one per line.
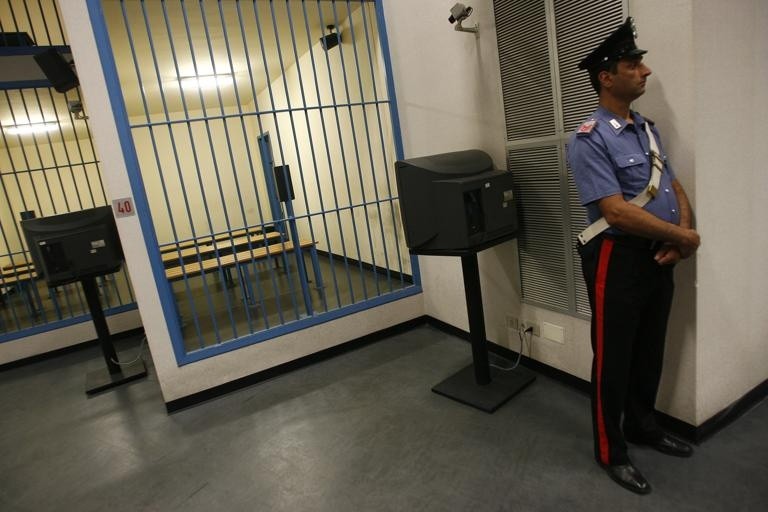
top-left (159, 225), bottom-right (329, 319)
top-left (0, 261), bottom-right (118, 342)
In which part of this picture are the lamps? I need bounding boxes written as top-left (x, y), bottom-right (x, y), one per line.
top-left (3, 120), bottom-right (60, 138)
top-left (174, 69), bottom-right (235, 93)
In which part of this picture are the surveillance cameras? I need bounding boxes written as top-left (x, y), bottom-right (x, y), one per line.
top-left (448, 3), bottom-right (470, 24)
top-left (319, 32), bottom-right (344, 51)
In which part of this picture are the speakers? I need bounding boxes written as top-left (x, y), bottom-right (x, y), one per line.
top-left (33, 47), bottom-right (80, 94)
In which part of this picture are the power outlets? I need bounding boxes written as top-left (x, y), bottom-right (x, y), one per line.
top-left (505, 315), bottom-right (565, 345)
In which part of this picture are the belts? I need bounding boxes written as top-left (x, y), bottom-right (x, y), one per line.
top-left (597, 224), bottom-right (665, 252)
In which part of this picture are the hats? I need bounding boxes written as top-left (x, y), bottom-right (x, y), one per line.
top-left (577, 15), bottom-right (649, 72)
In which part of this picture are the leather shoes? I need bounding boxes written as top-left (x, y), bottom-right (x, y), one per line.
top-left (593, 442), bottom-right (653, 496)
top-left (623, 427), bottom-right (694, 458)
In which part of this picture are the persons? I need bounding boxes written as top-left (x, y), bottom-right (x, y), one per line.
top-left (562, 14), bottom-right (702, 496)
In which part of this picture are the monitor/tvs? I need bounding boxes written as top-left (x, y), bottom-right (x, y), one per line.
top-left (20, 204), bottom-right (126, 289)
top-left (394, 149), bottom-right (520, 257)
